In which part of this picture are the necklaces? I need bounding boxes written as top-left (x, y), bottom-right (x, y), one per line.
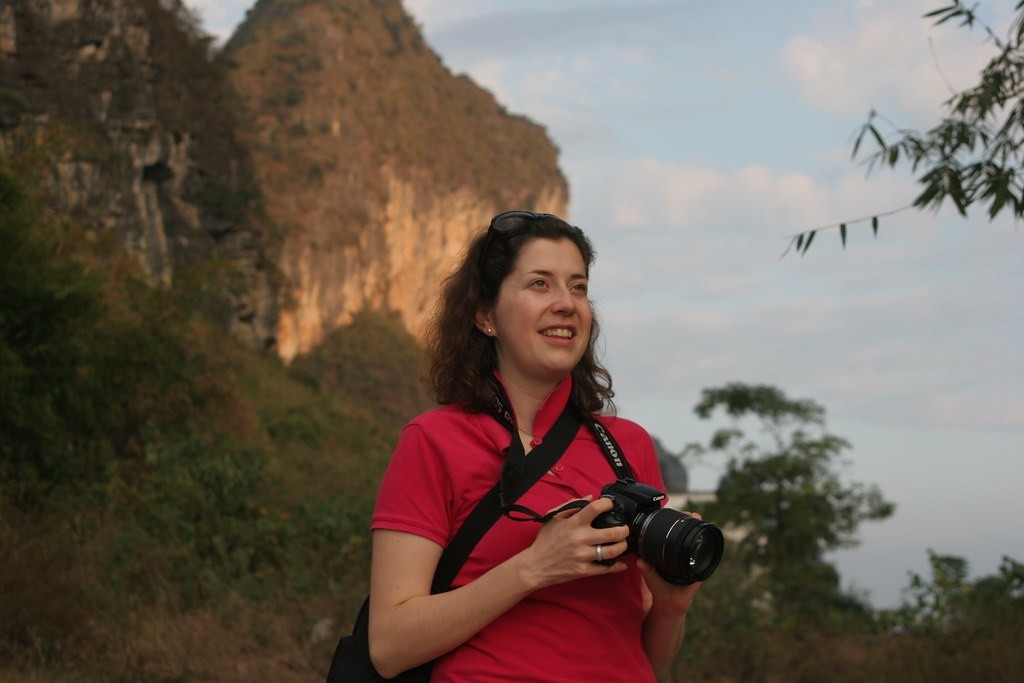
top-left (518, 427), bottom-right (536, 438)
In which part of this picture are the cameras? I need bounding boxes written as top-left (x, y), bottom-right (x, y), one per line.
top-left (590, 476), bottom-right (725, 582)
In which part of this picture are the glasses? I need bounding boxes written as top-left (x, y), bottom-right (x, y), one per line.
top-left (478, 210), bottom-right (560, 268)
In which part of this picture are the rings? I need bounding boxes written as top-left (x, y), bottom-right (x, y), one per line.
top-left (596, 545), bottom-right (601, 561)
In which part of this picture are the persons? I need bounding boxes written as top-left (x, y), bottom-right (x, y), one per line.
top-left (368, 213), bottom-right (703, 683)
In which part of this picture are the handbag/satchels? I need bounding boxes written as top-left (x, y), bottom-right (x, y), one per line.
top-left (324, 580), bottom-right (444, 683)
